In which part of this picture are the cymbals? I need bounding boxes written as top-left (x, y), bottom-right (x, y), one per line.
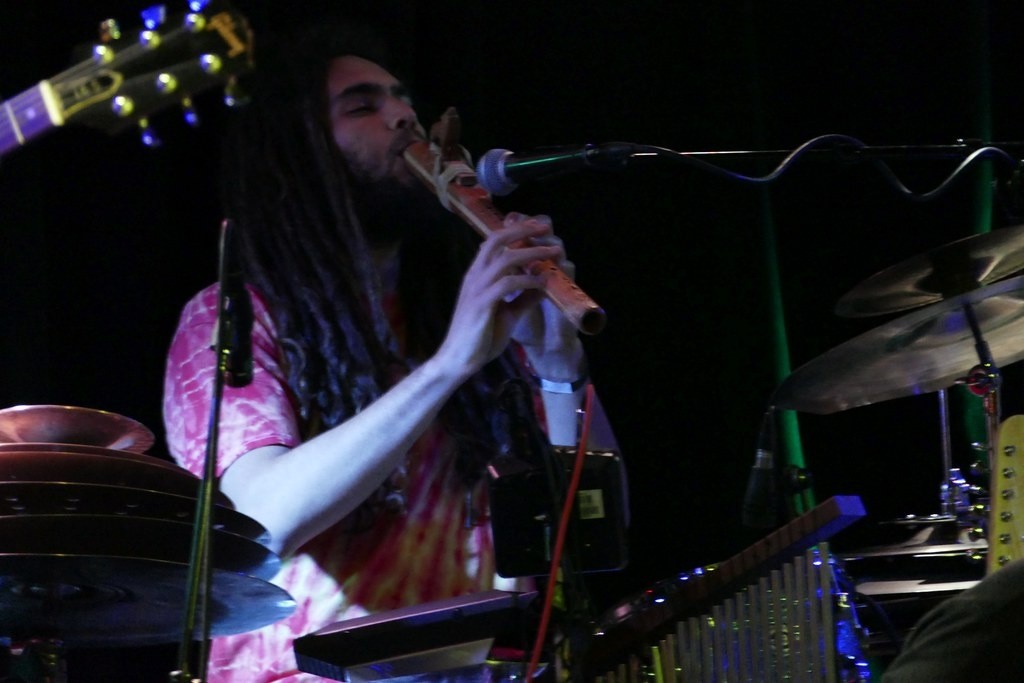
top-left (772, 277), bottom-right (1024, 420)
top-left (831, 220), bottom-right (1024, 324)
top-left (0, 403), bottom-right (297, 651)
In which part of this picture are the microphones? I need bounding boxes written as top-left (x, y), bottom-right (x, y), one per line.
top-left (221, 235), bottom-right (255, 388)
top-left (476, 140), bottom-right (634, 195)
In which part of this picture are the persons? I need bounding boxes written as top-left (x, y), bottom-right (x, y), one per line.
top-left (156, 35), bottom-right (633, 683)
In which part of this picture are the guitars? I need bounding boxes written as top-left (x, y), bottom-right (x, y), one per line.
top-left (0, 0), bottom-right (264, 164)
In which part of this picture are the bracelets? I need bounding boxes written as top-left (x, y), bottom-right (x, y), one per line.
top-left (523, 347), bottom-right (590, 394)
